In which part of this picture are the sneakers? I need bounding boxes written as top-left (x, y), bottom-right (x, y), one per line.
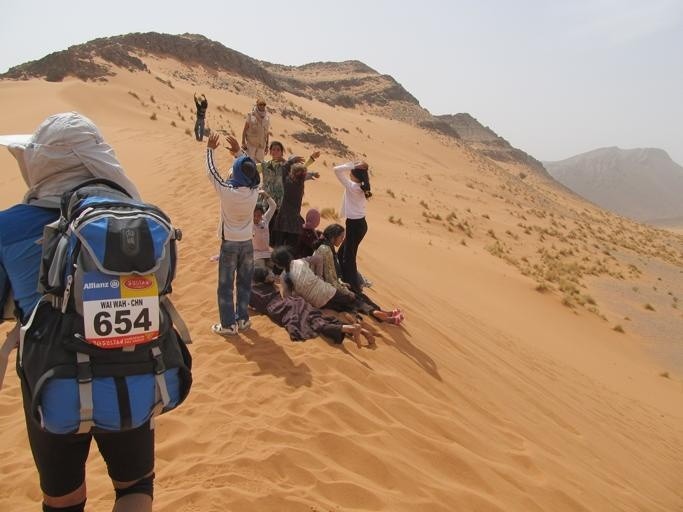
top-left (238, 318), bottom-right (253, 332)
top-left (210, 320), bottom-right (240, 337)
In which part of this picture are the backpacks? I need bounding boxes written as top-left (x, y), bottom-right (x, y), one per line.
top-left (10, 177), bottom-right (197, 438)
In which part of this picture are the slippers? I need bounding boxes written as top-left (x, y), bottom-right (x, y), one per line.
top-left (389, 307), bottom-right (400, 317)
top-left (394, 311), bottom-right (405, 327)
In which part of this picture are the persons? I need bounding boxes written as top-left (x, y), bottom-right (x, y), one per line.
top-left (240, 97), bottom-right (273, 164)
top-left (0, 111), bottom-right (159, 511)
top-left (192, 91), bottom-right (208, 143)
